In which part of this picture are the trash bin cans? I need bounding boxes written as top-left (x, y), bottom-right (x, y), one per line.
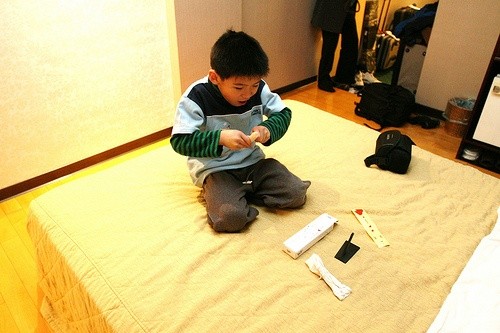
top-left (446, 97), bottom-right (472, 138)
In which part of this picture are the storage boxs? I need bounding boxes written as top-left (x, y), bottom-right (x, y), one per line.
top-left (283, 214), bottom-right (339, 259)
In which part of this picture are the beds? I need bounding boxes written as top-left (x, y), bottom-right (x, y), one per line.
top-left (25, 98), bottom-right (500, 333)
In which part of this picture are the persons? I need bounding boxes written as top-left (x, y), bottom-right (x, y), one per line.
top-left (311, 0), bottom-right (359, 92)
top-left (170, 31), bottom-right (311, 232)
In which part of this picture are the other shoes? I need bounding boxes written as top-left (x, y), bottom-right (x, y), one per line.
top-left (355, 71), bottom-right (364, 85)
top-left (362, 72), bottom-right (382, 83)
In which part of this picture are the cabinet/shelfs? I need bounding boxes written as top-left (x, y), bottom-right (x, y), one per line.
top-left (454, 32), bottom-right (499, 174)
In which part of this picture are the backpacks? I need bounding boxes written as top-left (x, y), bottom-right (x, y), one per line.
top-left (353, 82), bottom-right (415, 127)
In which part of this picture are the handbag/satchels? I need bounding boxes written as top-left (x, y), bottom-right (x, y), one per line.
top-left (365, 130), bottom-right (417, 174)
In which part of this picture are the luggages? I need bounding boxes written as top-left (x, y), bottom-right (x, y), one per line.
top-left (376, 35), bottom-right (401, 71)
